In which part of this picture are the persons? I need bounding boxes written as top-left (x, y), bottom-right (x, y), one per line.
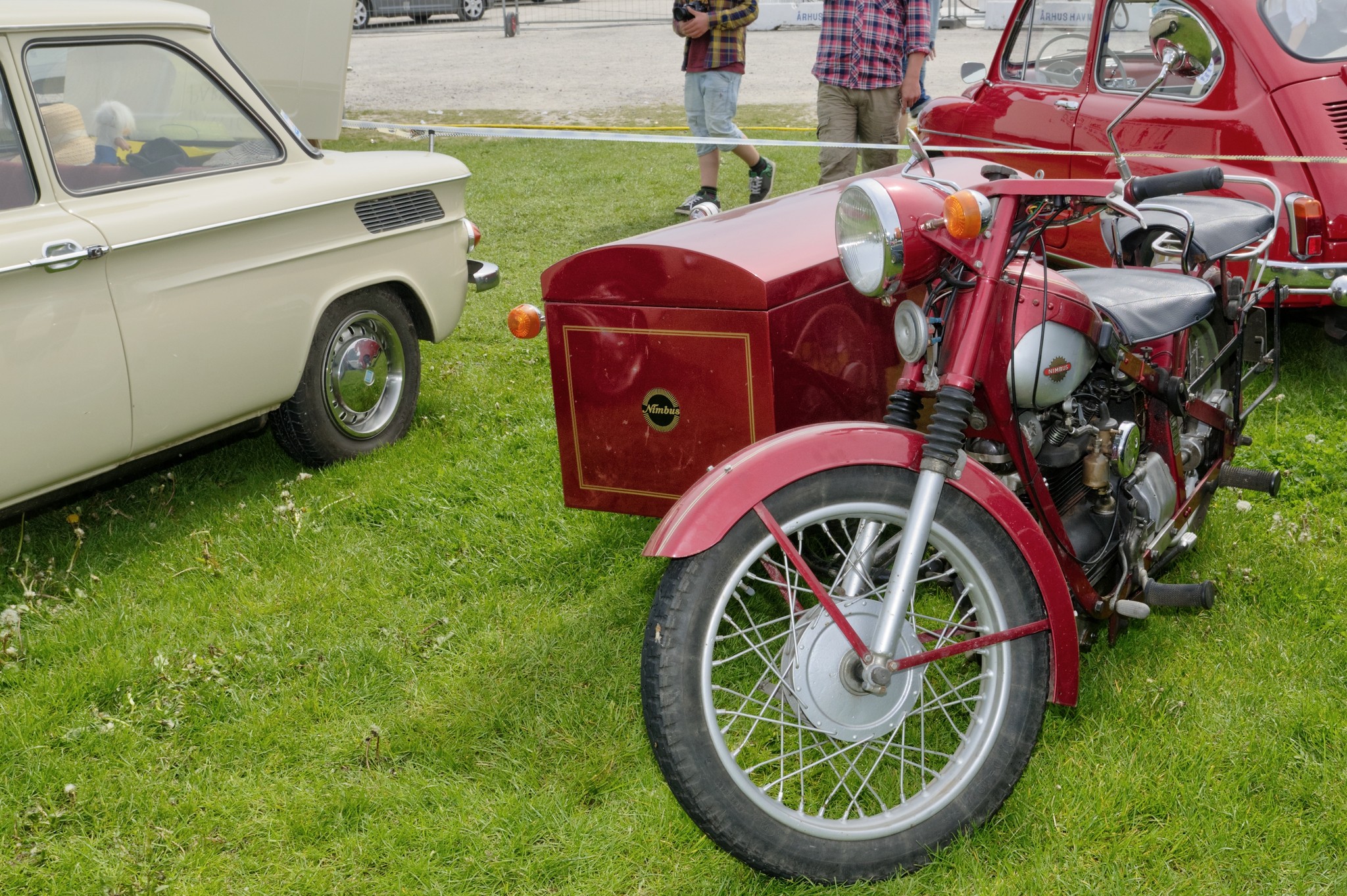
top-left (1265, 0), bottom-right (1347, 57)
top-left (673, 0), bottom-right (775, 215)
top-left (898, 0), bottom-right (942, 146)
top-left (811, 0), bottom-right (931, 186)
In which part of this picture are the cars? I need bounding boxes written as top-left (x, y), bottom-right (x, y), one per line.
top-left (353, 1), bottom-right (493, 29)
top-left (915, 1), bottom-right (1347, 310)
top-left (1, 1), bottom-right (501, 512)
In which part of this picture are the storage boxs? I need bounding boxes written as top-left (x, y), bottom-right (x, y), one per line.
top-left (539, 157), bottom-right (1036, 520)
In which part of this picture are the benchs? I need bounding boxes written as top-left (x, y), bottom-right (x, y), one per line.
top-left (1012, 61), bottom-right (1194, 87)
top-left (0, 161), bottom-right (209, 209)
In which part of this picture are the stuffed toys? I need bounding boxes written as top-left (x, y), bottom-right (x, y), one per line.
top-left (94, 101), bottom-right (136, 165)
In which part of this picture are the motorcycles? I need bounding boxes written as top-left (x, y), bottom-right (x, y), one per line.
top-left (642, 6), bottom-right (1282, 889)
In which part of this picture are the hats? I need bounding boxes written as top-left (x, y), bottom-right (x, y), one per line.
top-left (10, 102), bottom-right (96, 167)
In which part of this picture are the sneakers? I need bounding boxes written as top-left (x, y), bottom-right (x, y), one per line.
top-left (674, 191), bottom-right (721, 214)
top-left (749, 160), bottom-right (776, 203)
